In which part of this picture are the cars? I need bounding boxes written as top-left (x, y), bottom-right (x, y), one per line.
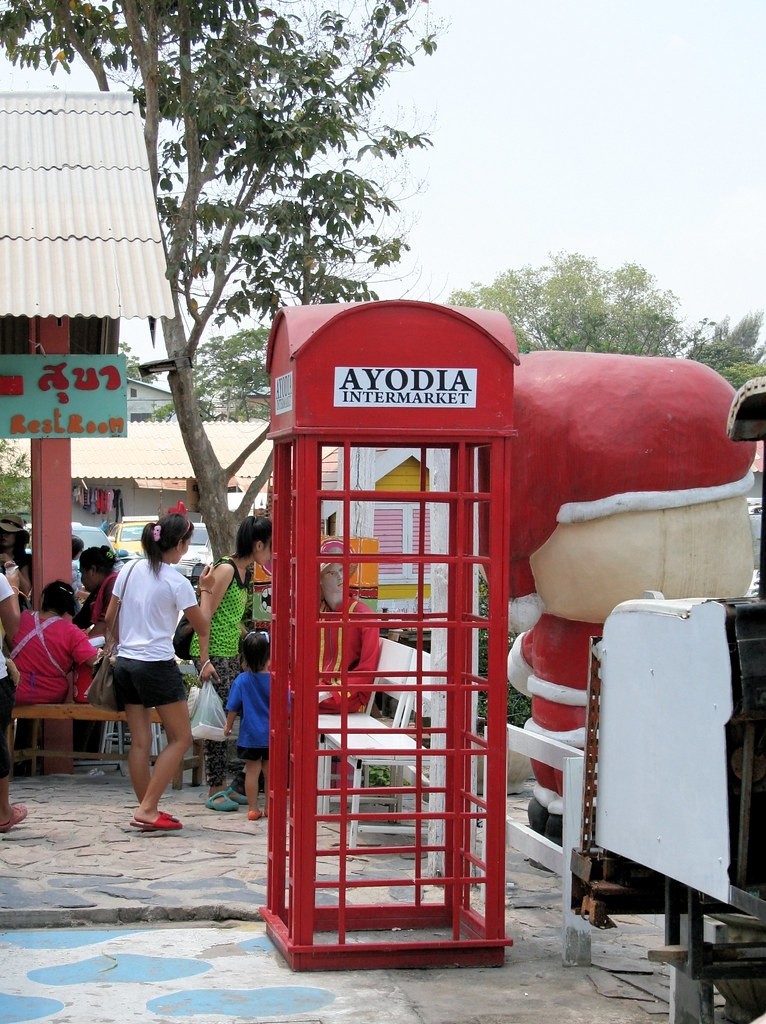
top-left (71, 522), bottom-right (130, 573)
top-left (170, 522), bottom-right (214, 578)
top-left (109, 514), bottom-right (160, 557)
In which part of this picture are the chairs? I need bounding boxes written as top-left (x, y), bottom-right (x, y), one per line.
top-left (317, 636), bottom-right (432, 857)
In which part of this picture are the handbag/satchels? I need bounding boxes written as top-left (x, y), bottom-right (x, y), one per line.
top-left (172, 559), bottom-right (236, 661)
top-left (186, 659), bottom-right (232, 742)
top-left (86, 656), bottom-right (117, 711)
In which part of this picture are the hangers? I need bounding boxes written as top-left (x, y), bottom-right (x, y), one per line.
top-left (72, 480), bottom-right (123, 497)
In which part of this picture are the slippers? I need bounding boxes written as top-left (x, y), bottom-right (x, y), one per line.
top-left (0, 805), bottom-right (28, 832)
top-left (226, 787), bottom-right (248, 805)
top-left (205, 790), bottom-right (239, 811)
top-left (130, 811), bottom-right (183, 832)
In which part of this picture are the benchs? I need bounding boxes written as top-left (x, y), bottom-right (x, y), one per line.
top-left (4, 695), bottom-right (208, 792)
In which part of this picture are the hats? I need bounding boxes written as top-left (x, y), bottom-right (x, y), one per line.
top-left (319, 563), bottom-right (331, 572)
top-left (0, 514), bottom-right (30, 544)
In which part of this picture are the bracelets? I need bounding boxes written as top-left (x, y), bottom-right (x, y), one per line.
top-left (197, 588), bottom-right (212, 594)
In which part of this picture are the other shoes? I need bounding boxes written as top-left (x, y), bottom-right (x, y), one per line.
top-left (258, 770), bottom-right (265, 791)
top-left (264, 810), bottom-right (267, 816)
top-left (248, 810), bottom-right (263, 820)
top-left (228, 770), bottom-right (246, 796)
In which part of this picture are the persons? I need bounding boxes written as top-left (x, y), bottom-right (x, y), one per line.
top-left (0, 500), bottom-right (291, 834)
top-left (318, 557), bottom-right (380, 714)
